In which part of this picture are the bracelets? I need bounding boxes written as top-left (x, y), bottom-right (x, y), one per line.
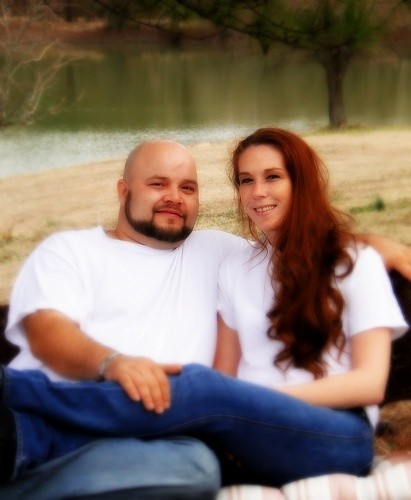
top-left (98, 350), bottom-right (119, 380)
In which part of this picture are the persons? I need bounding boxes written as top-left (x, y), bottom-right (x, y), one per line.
top-left (0, 127), bottom-right (409, 487)
top-left (0, 138), bottom-right (411, 500)
